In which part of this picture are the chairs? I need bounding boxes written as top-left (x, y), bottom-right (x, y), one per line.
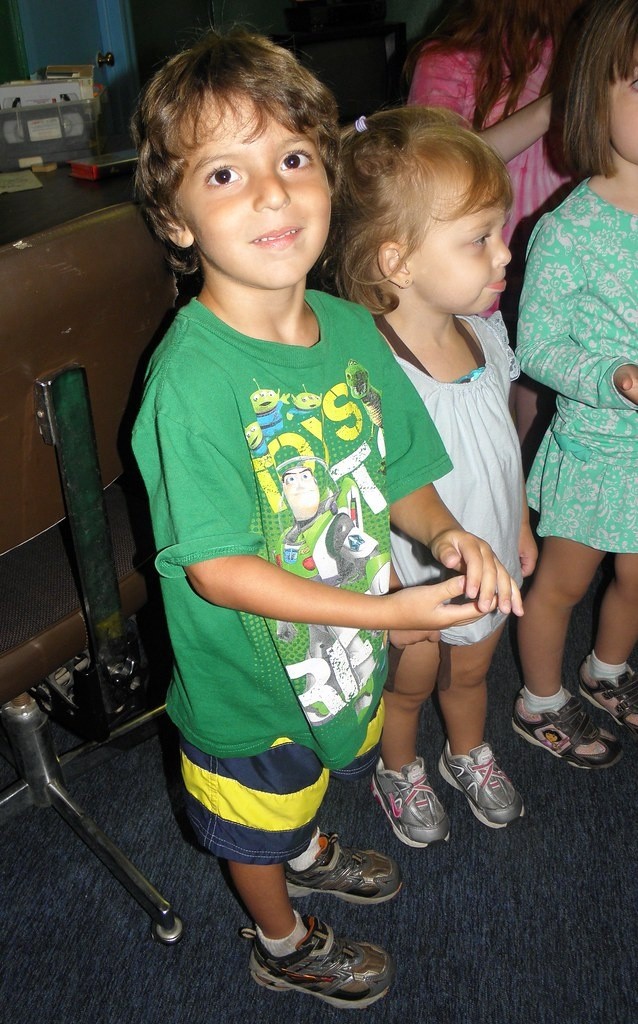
top-left (0, 201), bottom-right (184, 945)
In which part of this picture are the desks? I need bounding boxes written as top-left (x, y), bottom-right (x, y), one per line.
top-left (0, 134), bottom-right (137, 245)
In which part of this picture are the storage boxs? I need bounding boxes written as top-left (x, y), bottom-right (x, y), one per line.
top-left (0, 85), bottom-right (113, 172)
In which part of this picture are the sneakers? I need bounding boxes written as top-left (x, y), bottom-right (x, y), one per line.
top-left (579, 656), bottom-right (638, 731)
top-left (284, 833), bottom-right (402, 904)
top-left (241, 914), bottom-right (395, 1009)
top-left (438, 739), bottom-right (524, 829)
top-left (370, 755), bottom-right (448, 848)
top-left (512, 687), bottom-right (622, 769)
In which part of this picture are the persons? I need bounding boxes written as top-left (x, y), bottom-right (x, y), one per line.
top-left (511, 0), bottom-right (637, 769)
top-left (326, 107), bottom-right (539, 849)
top-left (408, 1), bottom-right (584, 475)
top-left (131, 33), bottom-right (525, 1009)
